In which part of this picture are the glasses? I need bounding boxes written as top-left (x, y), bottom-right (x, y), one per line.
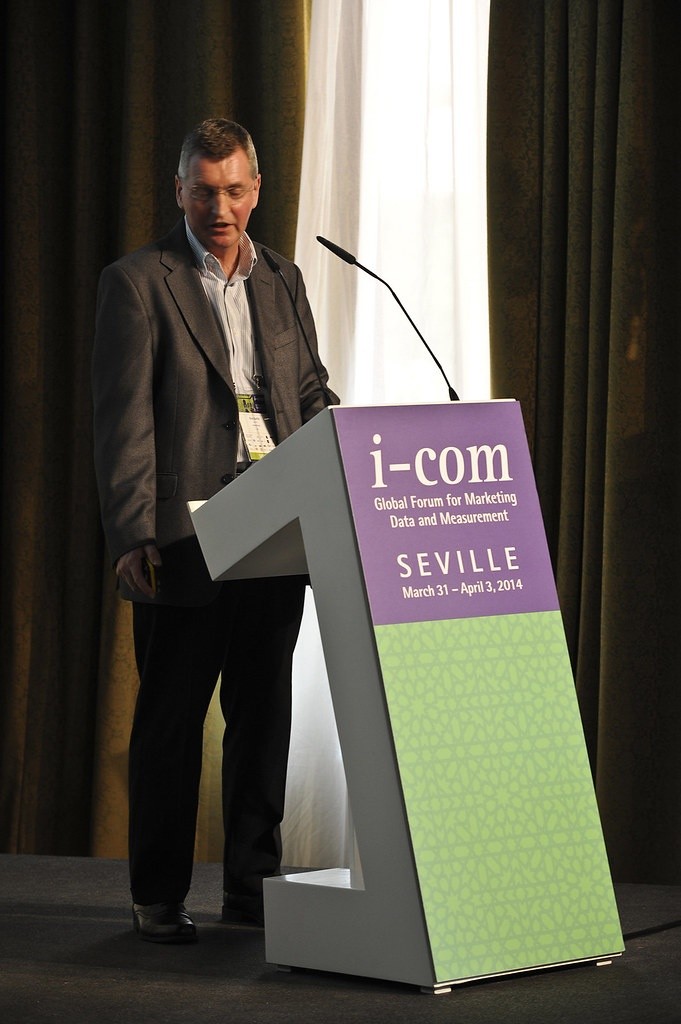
top-left (178, 176), bottom-right (256, 202)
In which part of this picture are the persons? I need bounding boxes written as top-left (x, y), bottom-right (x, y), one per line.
top-left (88, 117), bottom-right (342, 950)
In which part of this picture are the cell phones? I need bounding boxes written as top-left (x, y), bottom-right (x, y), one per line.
top-left (142, 557), bottom-right (158, 594)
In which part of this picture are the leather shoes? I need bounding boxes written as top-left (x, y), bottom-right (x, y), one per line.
top-left (132, 901), bottom-right (200, 943)
top-left (222, 891), bottom-right (264, 925)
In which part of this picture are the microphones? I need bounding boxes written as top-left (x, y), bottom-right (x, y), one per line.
top-left (316, 233), bottom-right (460, 403)
top-left (261, 247), bottom-right (333, 407)
